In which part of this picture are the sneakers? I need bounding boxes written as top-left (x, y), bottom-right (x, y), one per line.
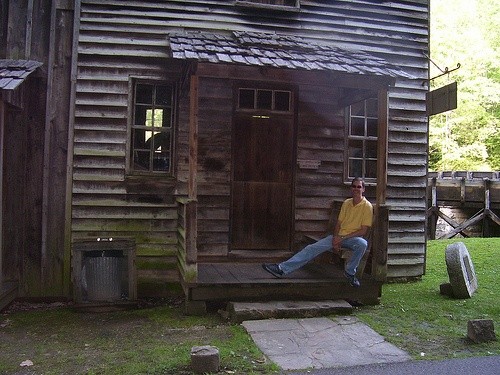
top-left (346, 274), bottom-right (360, 287)
top-left (263, 264), bottom-right (283, 279)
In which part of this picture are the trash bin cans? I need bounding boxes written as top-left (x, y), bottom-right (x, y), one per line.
top-left (67, 235), bottom-right (139, 312)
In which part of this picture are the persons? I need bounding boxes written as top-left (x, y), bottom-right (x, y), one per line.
top-left (261, 176), bottom-right (373, 288)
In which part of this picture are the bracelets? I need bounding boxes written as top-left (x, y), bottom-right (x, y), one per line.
top-left (341, 236), bottom-right (343, 239)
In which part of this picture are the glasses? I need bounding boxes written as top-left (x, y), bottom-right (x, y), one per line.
top-left (352, 186), bottom-right (362, 188)
